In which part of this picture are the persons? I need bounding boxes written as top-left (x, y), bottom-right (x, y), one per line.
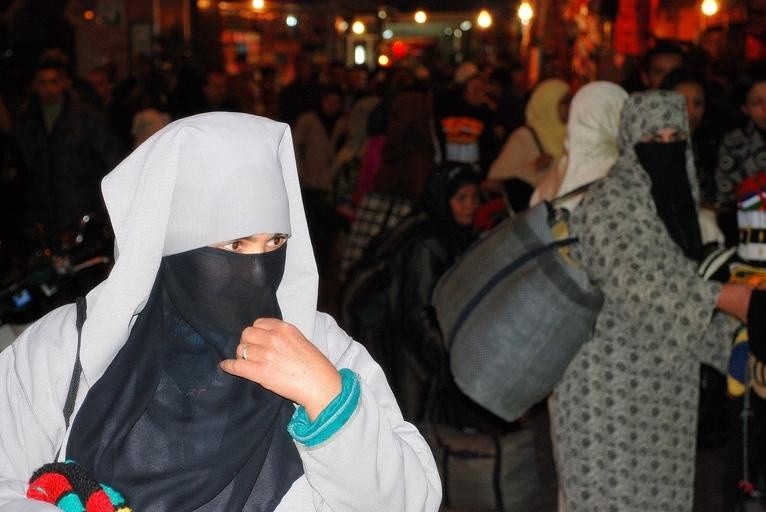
top-left (1, 0), bottom-right (765, 511)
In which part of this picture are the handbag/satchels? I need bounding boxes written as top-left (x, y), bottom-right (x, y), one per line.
top-left (431, 199), bottom-right (604, 424)
